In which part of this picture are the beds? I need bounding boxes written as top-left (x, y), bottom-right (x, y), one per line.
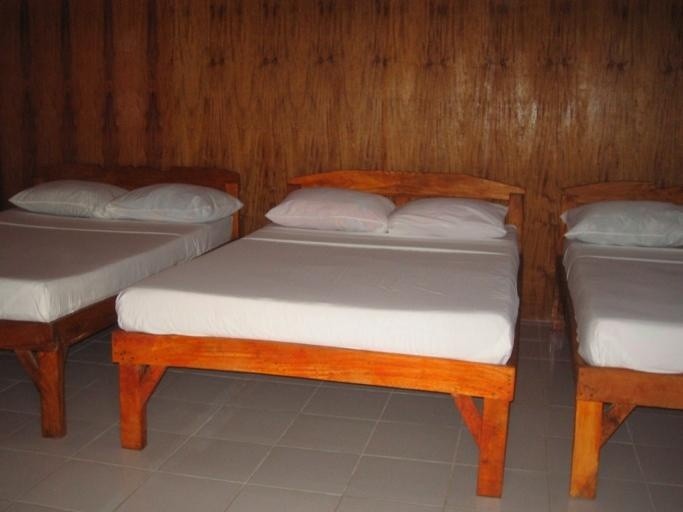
top-left (561, 180), bottom-right (683, 499)
top-left (0, 165), bottom-right (241, 438)
top-left (111, 168), bottom-right (536, 497)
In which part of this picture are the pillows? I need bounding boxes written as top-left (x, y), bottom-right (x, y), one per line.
top-left (106, 182), bottom-right (245, 222)
top-left (264, 187), bottom-right (395, 233)
top-left (389, 196), bottom-right (509, 240)
top-left (559, 199), bottom-right (683, 248)
top-left (8, 180), bottom-right (128, 217)
